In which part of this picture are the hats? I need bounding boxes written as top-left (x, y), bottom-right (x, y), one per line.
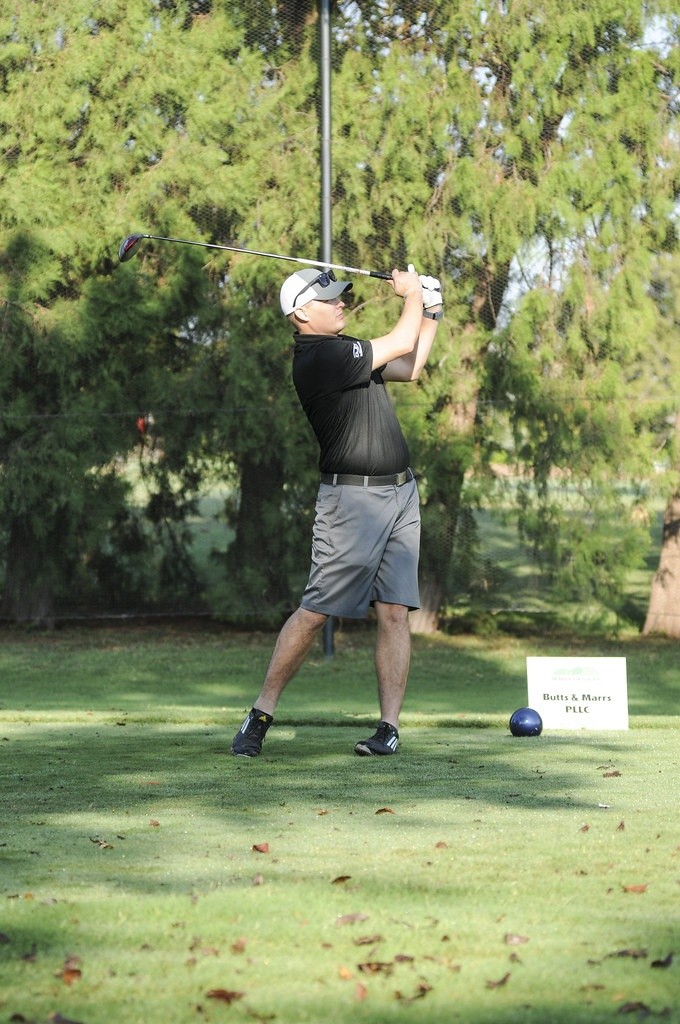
top-left (280, 268), bottom-right (353, 316)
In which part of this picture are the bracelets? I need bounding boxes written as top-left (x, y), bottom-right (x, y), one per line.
top-left (424, 310), bottom-right (443, 319)
top-left (404, 288), bottom-right (414, 301)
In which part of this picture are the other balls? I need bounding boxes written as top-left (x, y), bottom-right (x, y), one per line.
top-left (509, 707), bottom-right (543, 737)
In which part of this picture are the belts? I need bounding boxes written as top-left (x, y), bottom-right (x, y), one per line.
top-left (322, 467), bottom-right (414, 486)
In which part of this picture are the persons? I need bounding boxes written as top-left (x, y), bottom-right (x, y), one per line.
top-left (230, 264), bottom-right (442, 757)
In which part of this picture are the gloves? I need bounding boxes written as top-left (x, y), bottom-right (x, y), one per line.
top-left (407, 263), bottom-right (444, 310)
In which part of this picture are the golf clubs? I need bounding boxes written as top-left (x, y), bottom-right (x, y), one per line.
top-left (118, 232), bottom-right (441, 293)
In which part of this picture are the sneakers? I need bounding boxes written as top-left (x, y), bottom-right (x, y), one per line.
top-left (354, 722), bottom-right (399, 755)
top-left (232, 710), bottom-right (274, 758)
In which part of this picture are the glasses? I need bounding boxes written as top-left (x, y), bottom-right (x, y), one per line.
top-left (293, 270), bottom-right (337, 307)
